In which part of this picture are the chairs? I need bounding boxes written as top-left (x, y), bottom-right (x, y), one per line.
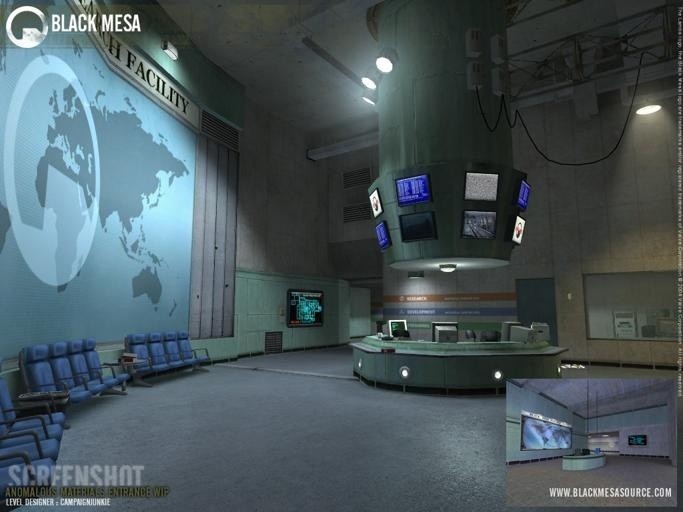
top-left (119, 329), bottom-right (218, 387)
top-left (0, 377), bottom-right (69, 511)
top-left (19, 337), bottom-right (133, 414)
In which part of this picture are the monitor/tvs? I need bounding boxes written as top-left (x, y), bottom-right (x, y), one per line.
top-left (288, 289), bottom-right (325, 327)
top-left (514, 177), bottom-right (532, 211)
top-left (368, 187), bottom-right (384, 219)
top-left (432, 321), bottom-right (459, 343)
top-left (382, 319), bottom-right (408, 338)
top-left (460, 210), bottom-right (498, 241)
top-left (511, 215), bottom-right (526, 245)
top-left (399, 211), bottom-right (439, 242)
top-left (628, 434), bottom-right (647, 445)
top-left (373, 220), bottom-right (392, 251)
top-left (463, 171), bottom-right (500, 204)
top-left (394, 172), bottom-right (434, 207)
top-left (502, 322), bottom-right (535, 342)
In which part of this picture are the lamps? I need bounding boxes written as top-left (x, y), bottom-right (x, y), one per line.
top-left (361, 46), bottom-right (399, 106)
